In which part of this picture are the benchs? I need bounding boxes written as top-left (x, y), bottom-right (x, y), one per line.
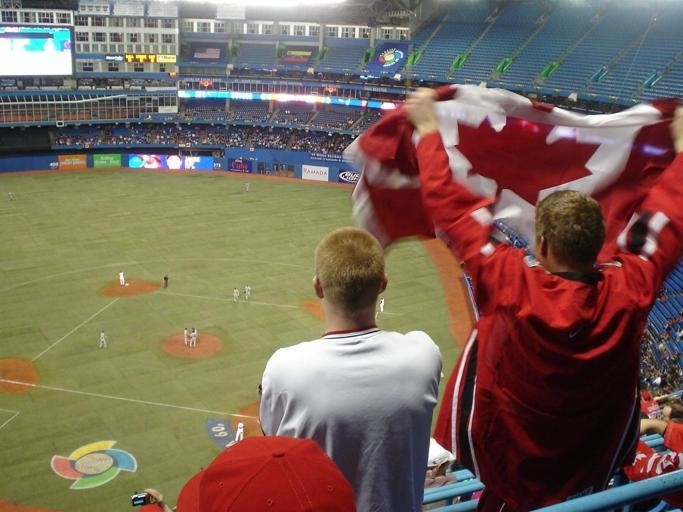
top-left (639, 259), bottom-right (683, 375)
top-left (489, 219), bottom-right (533, 258)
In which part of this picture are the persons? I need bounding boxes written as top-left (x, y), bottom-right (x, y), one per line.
top-left (603, 287), bottom-right (683, 511)
top-left (55, 114), bottom-right (178, 145)
top-left (98, 330), bottom-right (107, 348)
top-left (254, 224), bottom-right (442, 511)
top-left (179, 105), bottom-right (381, 152)
top-left (118, 270), bottom-right (124, 285)
top-left (191, 327), bottom-right (197, 342)
top-left (188, 330), bottom-right (195, 347)
top-left (182, 327), bottom-right (188, 346)
top-left (234, 420), bottom-right (244, 440)
top-left (419, 433), bottom-right (485, 511)
top-left (138, 488), bottom-right (174, 511)
top-left (162, 273), bottom-right (168, 288)
top-left (232, 287), bottom-right (239, 304)
top-left (395, 87), bottom-right (683, 511)
top-left (243, 284), bottom-right (251, 301)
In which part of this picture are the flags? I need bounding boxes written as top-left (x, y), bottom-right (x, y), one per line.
top-left (346, 89), bottom-right (683, 257)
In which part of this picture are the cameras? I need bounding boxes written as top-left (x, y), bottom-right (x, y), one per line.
top-left (130, 492), bottom-right (150, 507)
